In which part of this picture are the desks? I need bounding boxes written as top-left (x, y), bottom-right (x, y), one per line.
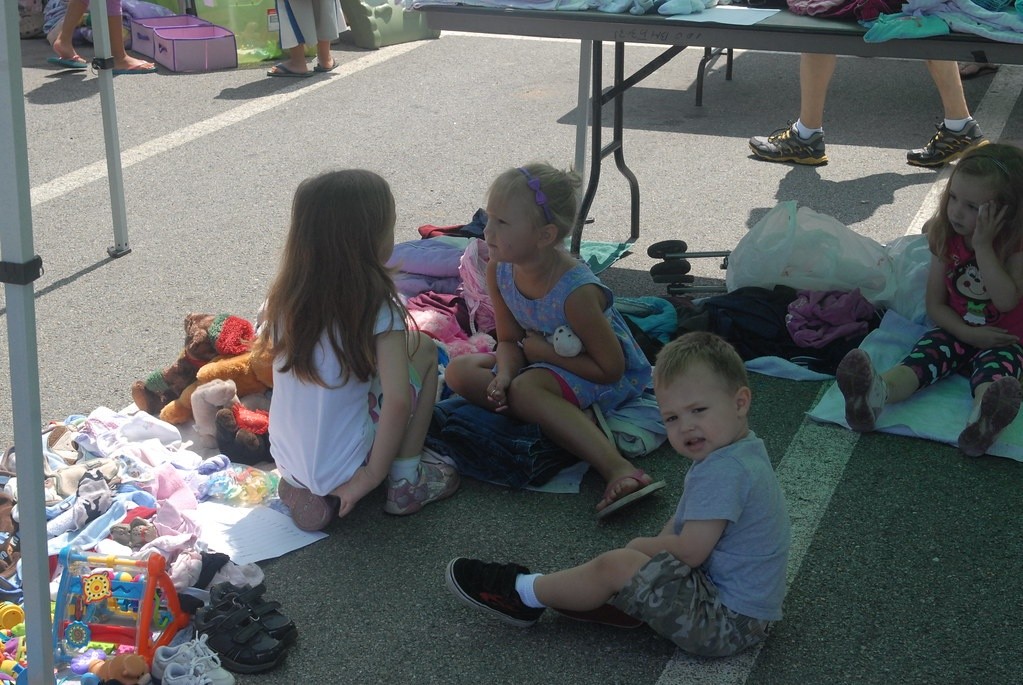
top-left (412, 0), bottom-right (1022, 255)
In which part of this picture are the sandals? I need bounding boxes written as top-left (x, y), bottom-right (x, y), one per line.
top-left (597, 470), bottom-right (667, 519)
top-left (582, 403), bottom-right (618, 451)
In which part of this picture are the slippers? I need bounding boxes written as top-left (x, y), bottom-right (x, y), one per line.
top-left (113, 60), bottom-right (158, 73)
top-left (268, 63), bottom-right (313, 76)
top-left (314, 59), bottom-right (339, 71)
top-left (48, 55), bottom-right (89, 68)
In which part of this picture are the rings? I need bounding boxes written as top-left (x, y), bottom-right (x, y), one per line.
top-left (490, 390), bottom-right (495, 396)
top-left (499, 401), bottom-right (502, 406)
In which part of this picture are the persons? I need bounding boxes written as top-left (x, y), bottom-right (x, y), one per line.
top-left (266, 0), bottom-right (351, 77)
top-left (748, 50), bottom-right (1023, 458)
top-left (443, 165), bottom-right (668, 515)
top-left (47, 0), bottom-right (157, 73)
top-left (444, 331), bottom-right (793, 658)
top-left (259, 164), bottom-right (460, 530)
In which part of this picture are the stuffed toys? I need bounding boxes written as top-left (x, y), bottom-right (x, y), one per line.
top-left (547, 326), bottom-right (588, 359)
top-left (133, 312), bottom-right (274, 467)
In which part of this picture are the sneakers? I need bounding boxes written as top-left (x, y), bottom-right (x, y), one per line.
top-left (278, 477), bottom-right (333, 530)
top-left (192, 582), bottom-right (298, 673)
top-left (837, 349), bottom-right (886, 433)
top-left (445, 558), bottom-right (546, 626)
top-left (959, 377), bottom-right (1020, 458)
top-left (387, 463), bottom-right (460, 515)
top-left (907, 120), bottom-right (989, 165)
top-left (553, 604), bottom-right (644, 628)
top-left (749, 120), bottom-right (828, 165)
top-left (151, 630), bottom-right (235, 685)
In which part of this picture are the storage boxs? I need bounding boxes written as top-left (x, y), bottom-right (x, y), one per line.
top-left (130, 14), bottom-right (239, 73)
